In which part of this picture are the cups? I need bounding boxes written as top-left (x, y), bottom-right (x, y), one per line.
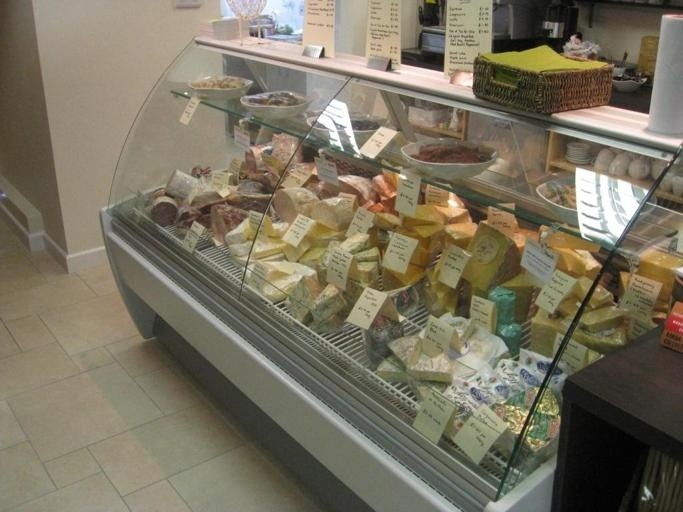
top-left (611, 67), bottom-right (626, 76)
top-left (594, 149), bottom-right (683, 197)
top-left (147, 170), bottom-right (199, 225)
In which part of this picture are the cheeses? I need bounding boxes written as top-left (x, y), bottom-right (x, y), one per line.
top-left (149, 135), bottom-right (682, 453)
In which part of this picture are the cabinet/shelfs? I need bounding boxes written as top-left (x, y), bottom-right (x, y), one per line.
top-left (95, 32), bottom-right (680, 512)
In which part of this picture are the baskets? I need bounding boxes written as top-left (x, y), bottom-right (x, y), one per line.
top-left (473, 44), bottom-right (616, 115)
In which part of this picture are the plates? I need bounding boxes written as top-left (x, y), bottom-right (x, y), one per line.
top-left (564, 142), bottom-right (593, 164)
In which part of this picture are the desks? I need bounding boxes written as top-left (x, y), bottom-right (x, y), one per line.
top-left (549, 323), bottom-right (682, 511)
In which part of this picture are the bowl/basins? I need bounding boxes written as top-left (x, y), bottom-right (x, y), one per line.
top-left (306, 114), bottom-right (396, 146)
top-left (611, 78), bottom-right (647, 92)
top-left (239, 90), bottom-right (310, 121)
top-left (186, 75), bottom-right (254, 101)
top-left (400, 139), bottom-right (499, 180)
top-left (534, 174), bottom-right (658, 230)
